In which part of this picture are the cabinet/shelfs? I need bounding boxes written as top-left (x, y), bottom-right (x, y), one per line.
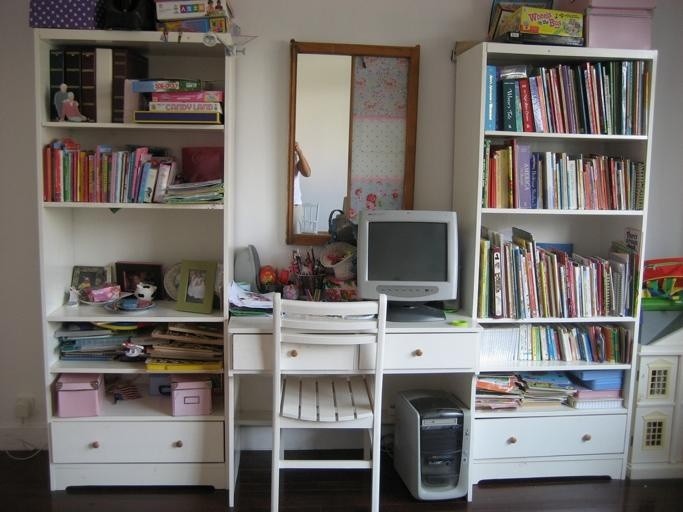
top-left (452, 43), bottom-right (658, 486)
top-left (34, 27), bottom-right (234, 491)
top-left (627, 355), bottom-right (683, 480)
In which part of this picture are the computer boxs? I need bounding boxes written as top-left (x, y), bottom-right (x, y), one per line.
top-left (399, 388), bottom-right (463, 492)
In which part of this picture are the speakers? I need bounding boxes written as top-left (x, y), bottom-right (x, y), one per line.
top-left (442, 297), bottom-right (459, 311)
top-left (234, 244), bottom-right (263, 294)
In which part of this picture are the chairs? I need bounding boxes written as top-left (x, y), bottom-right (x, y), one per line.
top-left (272, 293), bottom-right (387, 512)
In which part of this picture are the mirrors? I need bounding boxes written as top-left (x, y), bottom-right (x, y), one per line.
top-left (285, 38), bottom-right (421, 247)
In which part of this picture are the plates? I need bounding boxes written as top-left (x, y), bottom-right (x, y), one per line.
top-left (79, 295), bottom-right (117, 306)
top-left (104, 299), bottom-right (157, 314)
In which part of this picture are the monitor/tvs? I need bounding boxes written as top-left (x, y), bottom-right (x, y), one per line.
top-left (356, 209), bottom-right (459, 322)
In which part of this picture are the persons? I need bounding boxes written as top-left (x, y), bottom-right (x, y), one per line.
top-left (292, 141), bottom-right (312, 234)
top-left (194, 271), bottom-right (205, 300)
top-left (129, 274), bottom-right (142, 290)
top-left (77, 277), bottom-right (90, 288)
top-left (187, 270), bottom-right (196, 299)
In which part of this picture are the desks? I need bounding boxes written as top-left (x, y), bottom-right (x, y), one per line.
top-left (227, 306), bottom-right (485, 508)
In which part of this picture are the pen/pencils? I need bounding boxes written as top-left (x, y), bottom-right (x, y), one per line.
top-left (294, 248), bottom-right (324, 301)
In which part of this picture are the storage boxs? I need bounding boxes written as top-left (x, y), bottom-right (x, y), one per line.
top-left (55, 373), bottom-right (105, 418)
top-left (170, 376), bottom-right (213, 417)
top-left (29, 0), bottom-right (98, 29)
top-left (582, 7), bottom-right (653, 50)
top-left (557, 1), bottom-right (657, 13)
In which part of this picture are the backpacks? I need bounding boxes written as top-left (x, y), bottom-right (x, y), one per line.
top-left (643, 257), bottom-right (682, 299)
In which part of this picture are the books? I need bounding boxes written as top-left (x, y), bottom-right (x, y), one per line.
top-left (485, 59), bottom-right (649, 135)
top-left (43, 137), bottom-right (223, 214)
top-left (477, 226), bottom-right (642, 319)
top-left (482, 140), bottom-right (645, 210)
top-left (476, 371), bottom-right (624, 409)
top-left (478, 322), bottom-right (633, 364)
top-left (52, 321), bottom-right (225, 372)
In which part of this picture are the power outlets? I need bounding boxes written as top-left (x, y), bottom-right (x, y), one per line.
top-left (15, 394), bottom-right (38, 420)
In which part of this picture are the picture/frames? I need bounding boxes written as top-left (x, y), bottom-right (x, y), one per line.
top-left (71, 259), bottom-right (217, 314)
